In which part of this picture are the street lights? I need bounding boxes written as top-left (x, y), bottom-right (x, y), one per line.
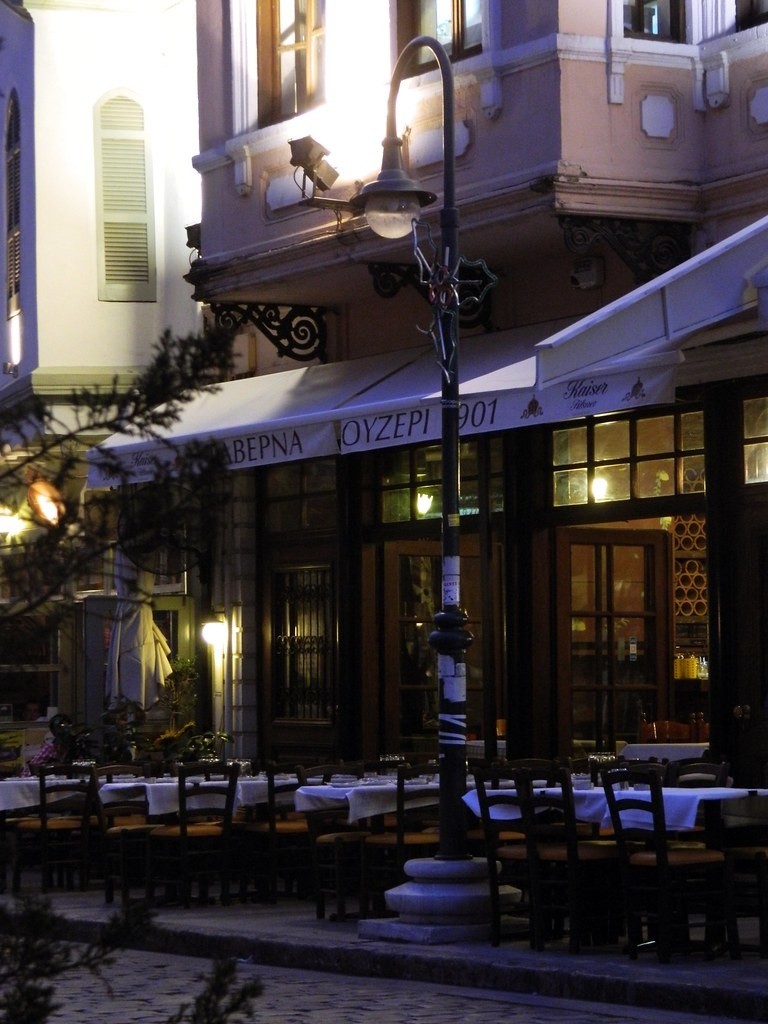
top-left (349, 36), bottom-right (523, 945)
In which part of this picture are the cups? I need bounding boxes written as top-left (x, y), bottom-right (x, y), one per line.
top-left (426, 759), bottom-right (438, 782)
top-left (379, 754), bottom-right (405, 778)
top-left (227, 759), bottom-right (252, 778)
top-left (587, 752), bottom-right (615, 768)
top-left (363, 772), bottom-right (378, 781)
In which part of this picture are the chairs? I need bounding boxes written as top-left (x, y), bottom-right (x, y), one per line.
top-left (0, 753), bottom-right (768, 963)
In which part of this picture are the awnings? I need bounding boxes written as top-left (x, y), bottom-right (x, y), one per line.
top-left (86, 215), bottom-right (768, 488)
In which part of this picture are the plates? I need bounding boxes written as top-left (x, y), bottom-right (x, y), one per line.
top-left (330, 780), bottom-right (427, 786)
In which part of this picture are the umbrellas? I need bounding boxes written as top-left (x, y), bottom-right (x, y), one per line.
top-left (104, 482), bottom-right (173, 758)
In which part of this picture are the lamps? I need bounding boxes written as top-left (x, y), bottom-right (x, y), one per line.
top-left (2, 362), bottom-right (18, 379)
top-left (415, 493), bottom-right (434, 520)
top-left (200, 608), bottom-right (230, 644)
top-left (288, 135), bottom-right (357, 214)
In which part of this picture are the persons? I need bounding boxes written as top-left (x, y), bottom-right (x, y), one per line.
top-left (21, 714), bottom-right (72, 778)
top-left (22, 703), bottom-right (40, 721)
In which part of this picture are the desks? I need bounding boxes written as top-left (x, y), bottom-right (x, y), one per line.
top-left (466, 739), bottom-right (710, 760)
top-left (294, 780), bottom-right (560, 920)
top-left (0, 776), bottom-right (204, 891)
top-left (98, 779), bottom-right (322, 903)
top-left (462, 787), bottom-right (767, 961)
